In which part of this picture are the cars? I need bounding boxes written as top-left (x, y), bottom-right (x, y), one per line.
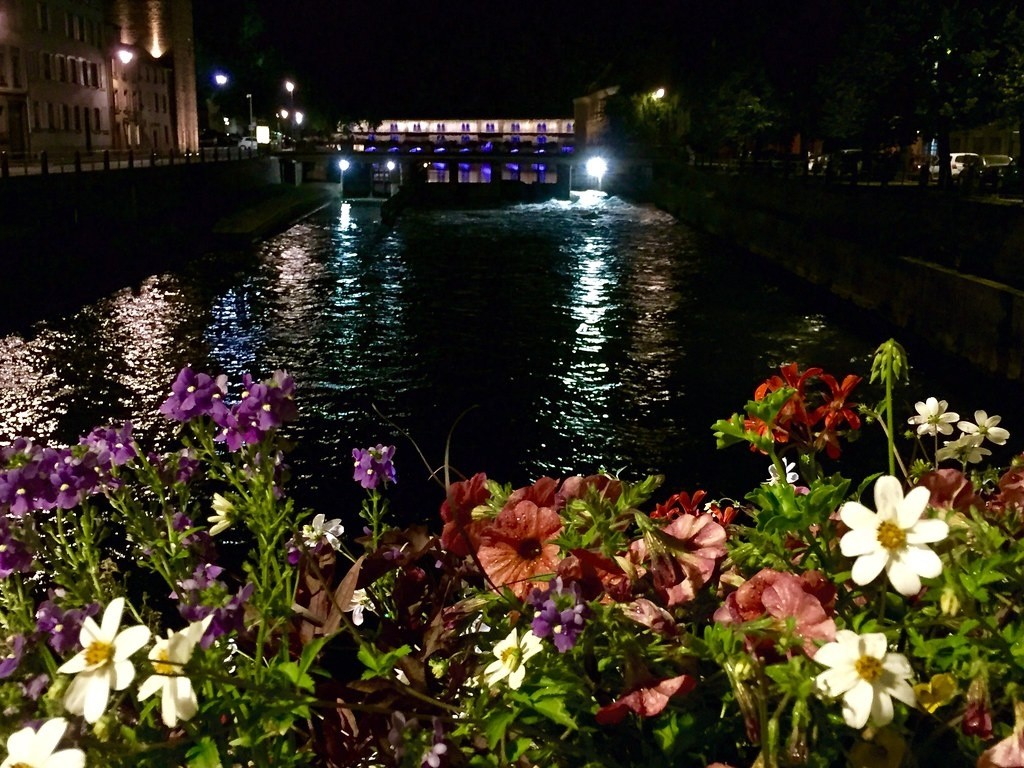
top-left (908, 152), bottom-right (1024, 185)
top-left (237, 136), bottom-right (258, 150)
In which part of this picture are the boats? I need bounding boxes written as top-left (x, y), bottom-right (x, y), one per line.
top-left (569, 190), bottom-right (610, 201)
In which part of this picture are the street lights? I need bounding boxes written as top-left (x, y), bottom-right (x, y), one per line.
top-left (246, 94), bottom-right (253, 150)
top-left (285, 80), bottom-right (296, 152)
